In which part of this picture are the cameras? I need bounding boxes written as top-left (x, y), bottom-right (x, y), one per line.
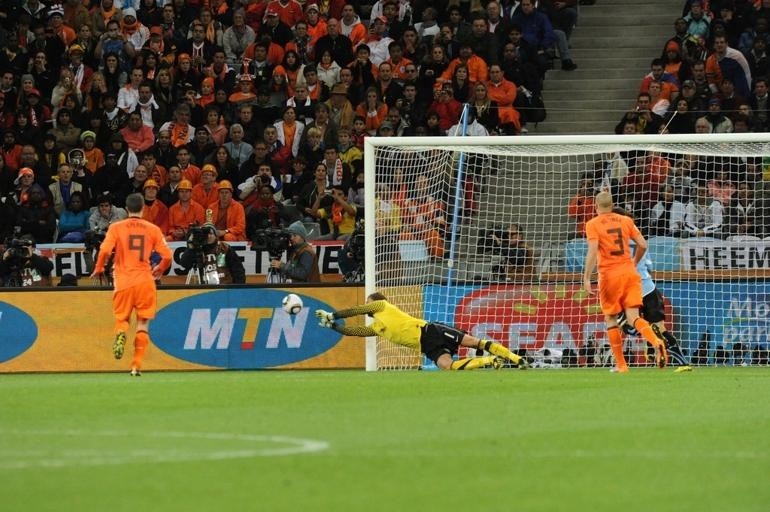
top-left (441, 91), bottom-right (446, 96)
top-left (260, 173), bottom-right (270, 183)
top-left (108, 31), bottom-right (117, 37)
top-left (400, 98), bottom-right (411, 106)
top-left (71, 158), bottom-right (80, 164)
top-left (323, 189), bottom-right (332, 197)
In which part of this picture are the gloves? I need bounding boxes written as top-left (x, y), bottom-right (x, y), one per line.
top-left (315, 310), bottom-right (333, 327)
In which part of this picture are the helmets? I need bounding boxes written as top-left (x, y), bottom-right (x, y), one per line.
top-left (143, 164), bottom-right (233, 191)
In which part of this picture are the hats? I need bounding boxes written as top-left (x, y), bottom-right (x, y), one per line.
top-left (330, 83), bottom-right (347, 94)
top-left (289, 221), bottom-right (307, 240)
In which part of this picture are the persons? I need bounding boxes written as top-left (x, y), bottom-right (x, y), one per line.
top-left (0, 2), bottom-right (582, 286)
top-left (558, 2), bottom-right (770, 287)
top-left (582, 193), bottom-right (668, 374)
top-left (90, 192), bottom-right (173, 376)
top-left (611, 207), bottom-right (693, 372)
top-left (316, 294), bottom-right (532, 372)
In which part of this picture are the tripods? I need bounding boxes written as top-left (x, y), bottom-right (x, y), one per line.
top-left (5, 269), bottom-right (23, 287)
top-left (92, 263), bottom-right (112, 285)
top-left (185, 262), bottom-right (207, 285)
top-left (264, 267), bottom-right (288, 284)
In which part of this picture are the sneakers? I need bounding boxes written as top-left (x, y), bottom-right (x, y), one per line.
top-left (130, 369), bottom-right (141, 376)
top-left (491, 323), bottom-right (689, 372)
top-left (113, 332), bottom-right (125, 359)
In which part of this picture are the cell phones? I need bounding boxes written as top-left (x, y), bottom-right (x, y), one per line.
top-left (370, 22), bottom-right (376, 34)
top-left (65, 77), bottom-right (69, 81)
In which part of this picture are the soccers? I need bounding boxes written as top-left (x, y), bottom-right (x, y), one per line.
top-left (282, 294), bottom-right (302, 314)
